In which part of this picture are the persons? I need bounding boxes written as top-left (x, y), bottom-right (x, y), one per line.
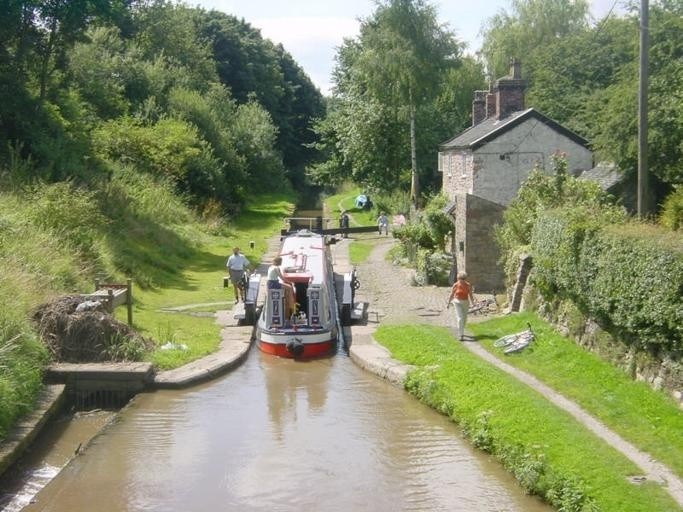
top-left (376, 211), bottom-right (389, 236)
top-left (266, 256), bottom-right (296, 315)
top-left (325, 219), bottom-right (332, 231)
top-left (398, 212), bottom-right (406, 227)
top-left (337, 214), bottom-right (344, 238)
top-left (341, 211), bottom-right (349, 238)
top-left (226, 247), bottom-right (253, 305)
top-left (444, 270), bottom-right (478, 342)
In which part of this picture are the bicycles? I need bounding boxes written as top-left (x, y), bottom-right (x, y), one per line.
top-left (492, 321), bottom-right (537, 354)
top-left (464, 288), bottom-right (499, 314)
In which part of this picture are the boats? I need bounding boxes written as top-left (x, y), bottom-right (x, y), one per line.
top-left (253, 228), bottom-right (343, 362)
